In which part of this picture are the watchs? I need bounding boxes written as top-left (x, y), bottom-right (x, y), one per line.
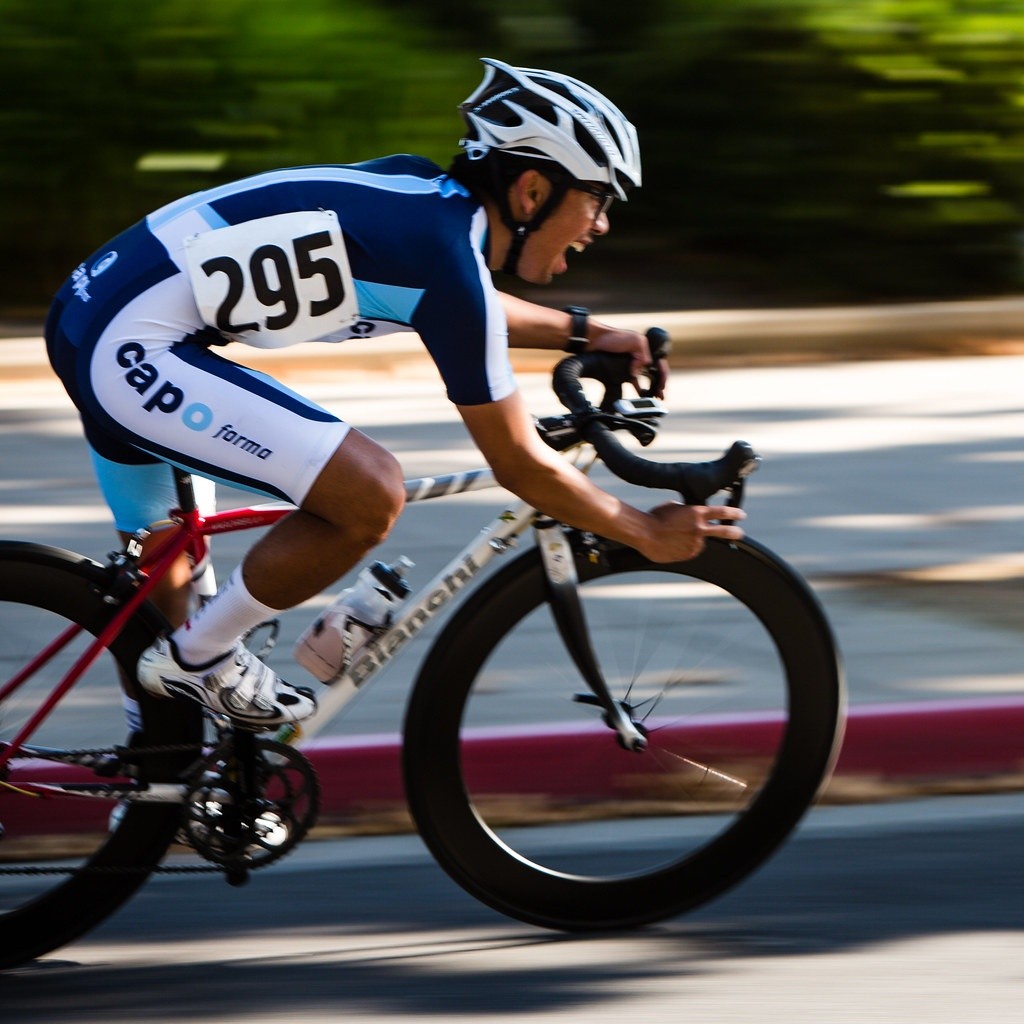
top-left (564, 304), bottom-right (591, 353)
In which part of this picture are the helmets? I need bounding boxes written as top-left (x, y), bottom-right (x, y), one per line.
top-left (457, 58), bottom-right (642, 202)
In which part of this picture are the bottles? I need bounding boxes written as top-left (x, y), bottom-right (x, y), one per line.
top-left (295, 552), bottom-right (414, 688)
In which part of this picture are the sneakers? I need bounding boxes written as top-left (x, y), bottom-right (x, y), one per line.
top-left (137, 635), bottom-right (319, 726)
top-left (108, 796), bottom-right (288, 852)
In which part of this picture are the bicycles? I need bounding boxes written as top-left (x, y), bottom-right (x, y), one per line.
top-left (0, 326), bottom-right (851, 972)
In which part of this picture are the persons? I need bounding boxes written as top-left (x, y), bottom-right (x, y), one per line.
top-left (40, 51), bottom-right (747, 857)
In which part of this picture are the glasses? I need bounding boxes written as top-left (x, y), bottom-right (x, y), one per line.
top-left (570, 180), bottom-right (615, 225)
